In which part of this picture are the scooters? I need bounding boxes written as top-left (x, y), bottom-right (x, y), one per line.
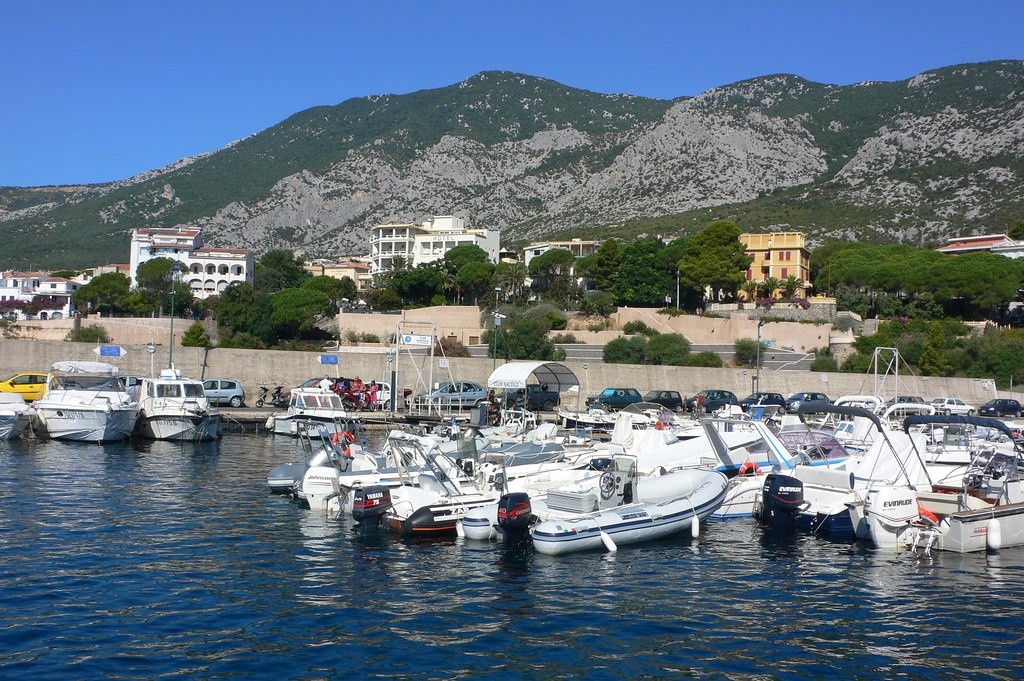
top-left (341, 386), bottom-right (376, 413)
top-left (255, 385), bottom-right (296, 410)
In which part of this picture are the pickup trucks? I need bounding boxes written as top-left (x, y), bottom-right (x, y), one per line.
top-left (493, 383), bottom-right (560, 412)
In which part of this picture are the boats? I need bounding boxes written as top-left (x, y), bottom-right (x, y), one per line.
top-left (30, 336), bottom-right (143, 445)
top-left (0, 391), bottom-right (38, 441)
top-left (139, 361), bottom-right (224, 442)
top-left (266, 401), bottom-right (1024, 556)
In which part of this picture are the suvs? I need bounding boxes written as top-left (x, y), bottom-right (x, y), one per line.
top-left (281, 377), bottom-right (355, 407)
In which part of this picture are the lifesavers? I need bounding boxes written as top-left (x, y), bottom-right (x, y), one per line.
top-left (331, 432), bottom-right (356, 457)
top-left (914, 507), bottom-right (940, 525)
top-left (740, 462), bottom-right (764, 477)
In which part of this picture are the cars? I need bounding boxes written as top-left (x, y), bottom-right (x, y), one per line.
top-left (977, 398), bottom-right (1022, 418)
top-left (785, 392), bottom-right (831, 415)
top-left (361, 382), bottom-right (391, 411)
top-left (174, 378), bottom-right (246, 408)
top-left (683, 389), bottom-right (738, 414)
top-left (738, 391), bottom-right (787, 415)
top-left (929, 397), bottom-right (976, 417)
top-left (830, 394), bottom-right (888, 415)
top-left (413, 379), bottom-right (488, 410)
top-left (884, 395), bottom-right (926, 415)
top-left (0, 371), bottom-right (63, 401)
top-left (584, 386), bottom-right (642, 411)
top-left (643, 390), bottom-right (684, 413)
top-left (85, 374), bottom-right (150, 403)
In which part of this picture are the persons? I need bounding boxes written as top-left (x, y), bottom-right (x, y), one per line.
top-left (696, 307), bottom-right (703, 315)
top-left (370, 380), bottom-right (378, 411)
top-left (336, 376), bottom-right (366, 392)
top-left (319, 375), bottom-right (333, 389)
top-left (489, 390), bottom-right (498, 402)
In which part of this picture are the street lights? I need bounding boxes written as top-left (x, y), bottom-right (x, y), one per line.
top-left (493, 284), bottom-right (502, 371)
top-left (756, 318), bottom-right (768, 393)
top-left (167, 263), bottom-right (181, 368)
top-left (675, 270), bottom-right (680, 311)
